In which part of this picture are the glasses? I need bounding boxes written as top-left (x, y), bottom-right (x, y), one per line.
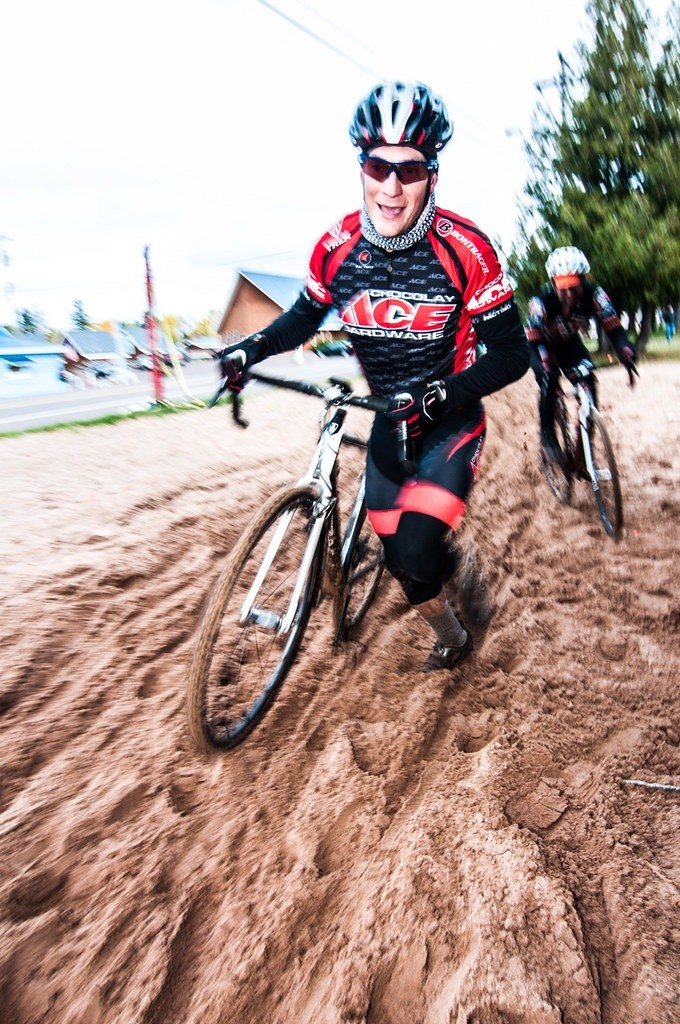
top-left (358, 152), bottom-right (438, 186)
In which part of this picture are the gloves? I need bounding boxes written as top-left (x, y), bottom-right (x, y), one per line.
top-left (383, 379), bottom-right (451, 442)
top-left (212, 333), bottom-right (269, 393)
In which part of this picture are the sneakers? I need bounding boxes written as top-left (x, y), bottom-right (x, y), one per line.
top-left (422, 625), bottom-right (473, 672)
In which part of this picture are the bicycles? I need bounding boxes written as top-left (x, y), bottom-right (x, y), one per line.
top-left (188, 370), bottom-right (386, 756)
top-left (536, 353), bottom-right (634, 544)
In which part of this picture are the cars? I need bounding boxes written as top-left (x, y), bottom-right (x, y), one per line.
top-left (0, 324), bottom-right (238, 395)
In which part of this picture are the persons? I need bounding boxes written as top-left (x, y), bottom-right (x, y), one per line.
top-left (524, 243), bottom-right (638, 484)
top-left (218, 81), bottom-right (533, 672)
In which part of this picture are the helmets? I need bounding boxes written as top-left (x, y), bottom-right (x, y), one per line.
top-left (349, 77), bottom-right (454, 153)
top-left (544, 245), bottom-right (591, 290)
